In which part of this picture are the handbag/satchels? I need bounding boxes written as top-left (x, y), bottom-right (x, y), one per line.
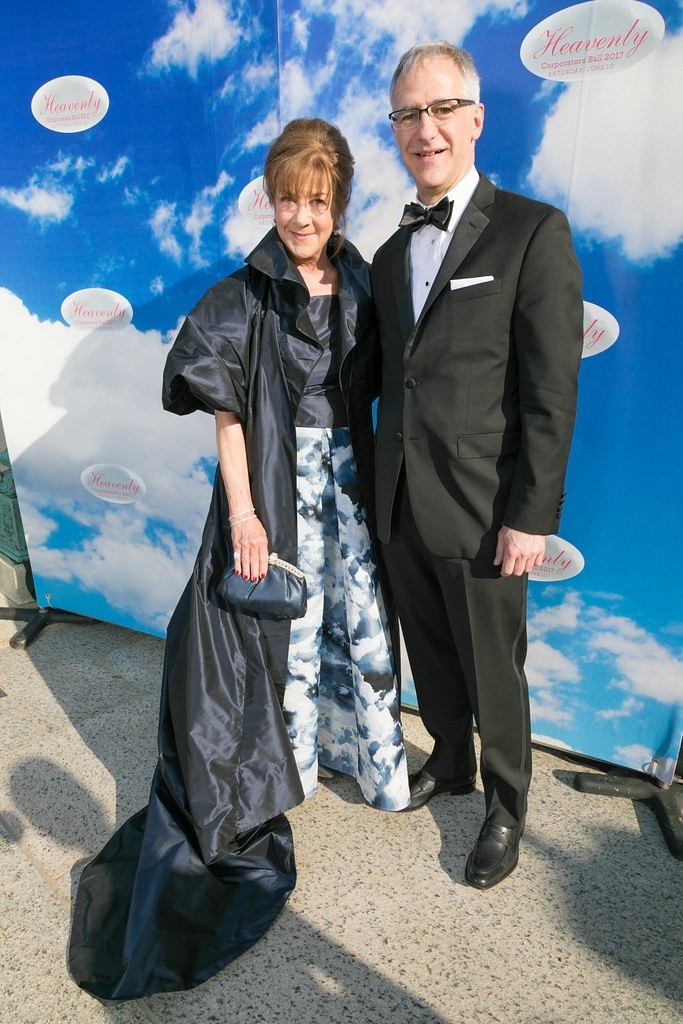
top-left (216, 553), bottom-right (307, 620)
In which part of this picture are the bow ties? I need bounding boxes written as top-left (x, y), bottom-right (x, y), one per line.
top-left (398, 196), bottom-right (454, 234)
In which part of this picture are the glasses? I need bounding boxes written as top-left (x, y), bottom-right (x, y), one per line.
top-left (389, 98), bottom-right (475, 130)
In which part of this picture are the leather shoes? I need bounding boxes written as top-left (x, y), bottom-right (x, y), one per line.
top-left (465, 815), bottom-right (525, 890)
top-left (399, 768), bottom-right (476, 812)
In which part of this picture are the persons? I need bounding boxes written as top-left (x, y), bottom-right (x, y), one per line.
top-left (372, 42), bottom-right (584, 890)
top-left (65, 119), bottom-right (414, 1001)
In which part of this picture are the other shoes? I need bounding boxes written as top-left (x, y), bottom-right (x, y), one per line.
top-left (317, 764), bottom-right (334, 778)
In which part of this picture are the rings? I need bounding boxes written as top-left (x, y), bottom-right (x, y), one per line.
top-left (233, 552), bottom-right (241, 559)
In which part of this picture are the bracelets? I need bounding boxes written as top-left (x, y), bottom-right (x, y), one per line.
top-left (228, 508), bottom-right (255, 521)
top-left (230, 515), bottom-right (257, 527)
